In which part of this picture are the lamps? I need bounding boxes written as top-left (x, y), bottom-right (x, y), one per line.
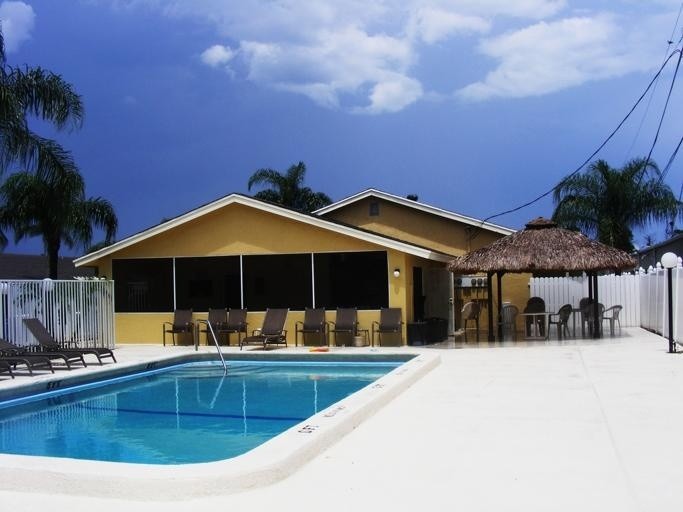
top-left (393, 267), bottom-right (399, 277)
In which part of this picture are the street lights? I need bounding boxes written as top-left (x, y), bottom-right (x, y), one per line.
top-left (0, 282), bottom-right (10, 341)
top-left (659, 251), bottom-right (680, 353)
top-left (41, 278), bottom-right (54, 334)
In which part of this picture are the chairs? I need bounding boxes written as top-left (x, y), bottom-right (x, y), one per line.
top-left (461, 297), bottom-right (623, 341)
top-left (0, 318), bottom-right (117, 379)
top-left (162, 306), bottom-right (404, 351)
